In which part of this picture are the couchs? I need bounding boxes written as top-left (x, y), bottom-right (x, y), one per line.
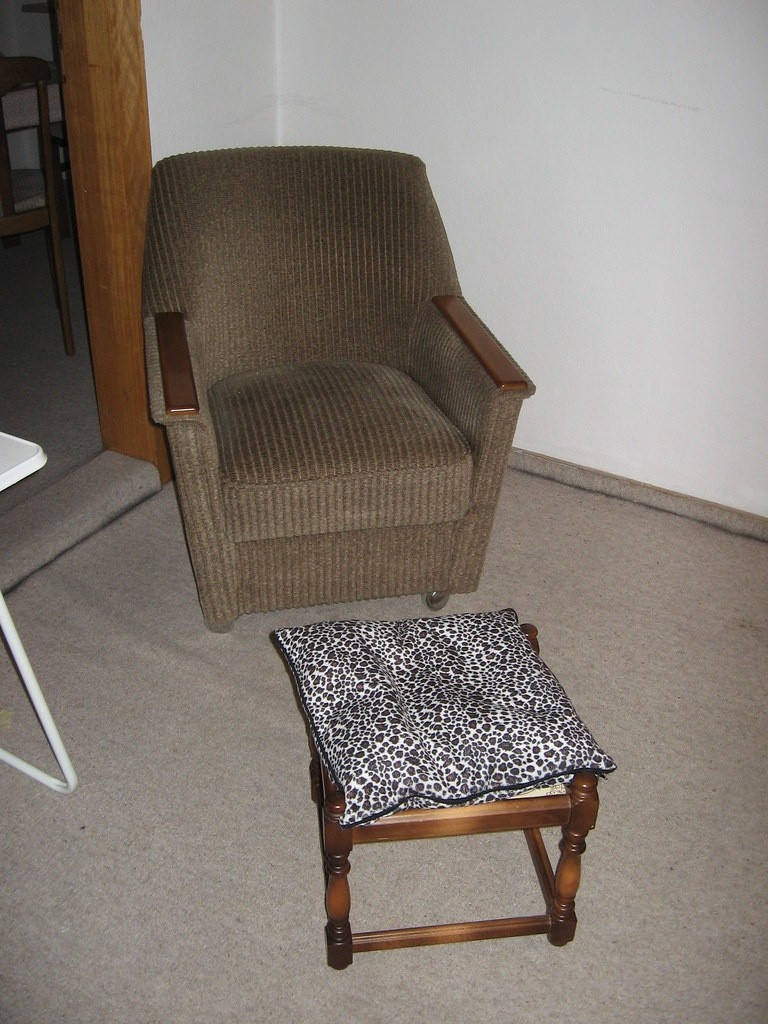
top-left (138, 147), bottom-right (538, 633)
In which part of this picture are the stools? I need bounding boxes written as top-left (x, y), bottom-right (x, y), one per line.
top-left (306, 624), bottom-right (602, 974)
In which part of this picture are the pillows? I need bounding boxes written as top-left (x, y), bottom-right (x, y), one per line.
top-left (268, 606), bottom-right (617, 828)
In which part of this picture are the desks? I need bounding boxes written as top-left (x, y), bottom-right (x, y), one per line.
top-left (0, 434), bottom-right (77, 790)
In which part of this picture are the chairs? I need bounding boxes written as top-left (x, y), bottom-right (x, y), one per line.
top-left (1, 54), bottom-right (75, 356)
